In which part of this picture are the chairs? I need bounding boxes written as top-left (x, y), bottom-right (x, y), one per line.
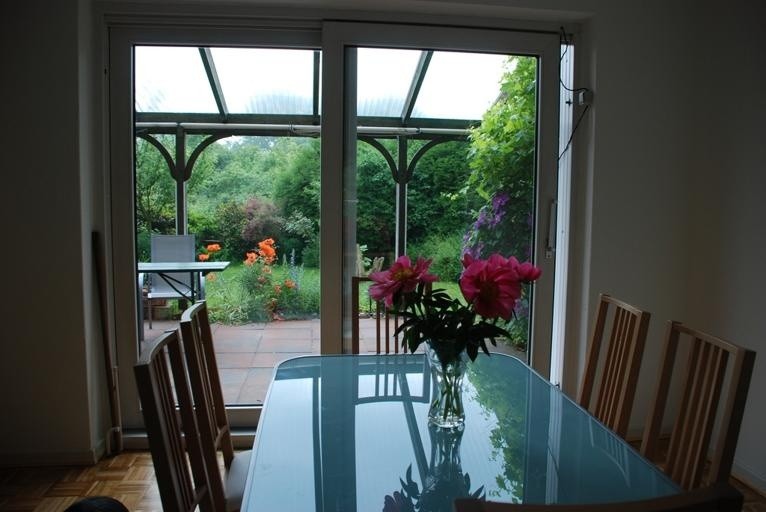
top-left (147, 234), bottom-right (195, 328)
top-left (576, 292), bottom-right (653, 439)
top-left (179, 301), bottom-right (253, 504)
top-left (133, 328), bottom-right (238, 512)
top-left (353, 277), bottom-right (436, 356)
top-left (639, 316), bottom-right (756, 493)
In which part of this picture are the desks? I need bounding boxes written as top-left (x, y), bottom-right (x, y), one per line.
top-left (239, 352), bottom-right (686, 511)
top-left (139, 262), bottom-right (233, 340)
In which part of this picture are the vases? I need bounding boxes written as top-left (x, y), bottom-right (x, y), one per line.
top-left (424, 342), bottom-right (468, 430)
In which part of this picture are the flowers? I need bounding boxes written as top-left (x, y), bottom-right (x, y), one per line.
top-left (370, 256), bottom-right (542, 424)
